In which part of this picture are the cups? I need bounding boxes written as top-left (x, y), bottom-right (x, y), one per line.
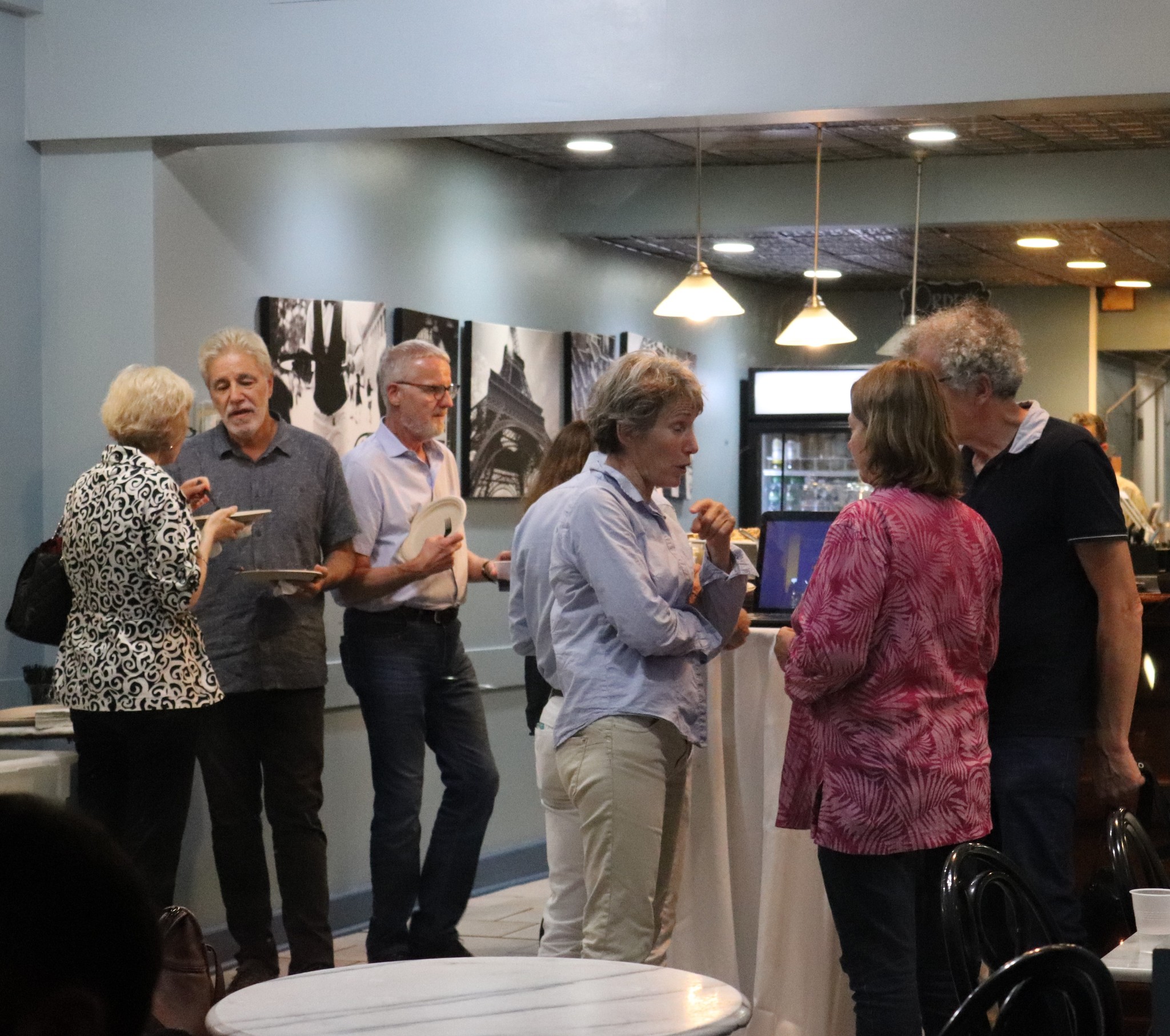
top-left (1153, 522), bottom-right (1170, 551)
top-left (495, 561), bottom-right (510, 592)
top-left (1129, 888), bottom-right (1170, 954)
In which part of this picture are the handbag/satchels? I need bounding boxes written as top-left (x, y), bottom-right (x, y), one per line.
top-left (4, 508), bottom-right (75, 645)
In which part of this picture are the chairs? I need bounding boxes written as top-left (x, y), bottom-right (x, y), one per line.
top-left (938, 841), bottom-right (1060, 997)
top-left (936, 942), bottom-right (1123, 1036)
top-left (1106, 802), bottom-right (1170, 916)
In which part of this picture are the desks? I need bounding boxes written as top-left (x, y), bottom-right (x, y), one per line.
top-left (203, 954), bottom-right (756, 1036)
top-left (684, 624), bottom-right (857, 1036)
top-left (1098, 933), bottom-right (1170, 985)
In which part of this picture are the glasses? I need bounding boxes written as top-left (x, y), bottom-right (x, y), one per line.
top-left (394, 381), bottom-right (461, 401)
top-left (184, 425), bottom-right (196, 439)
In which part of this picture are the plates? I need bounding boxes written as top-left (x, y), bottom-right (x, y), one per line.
top-left (236, 569), bottom-right (323, 582)
top-left (746, 582), bottom-right (756, 592)
top-left (193, 509), bottom-right (272, 527)
top-left (400, 496), bottom-right (467, 562)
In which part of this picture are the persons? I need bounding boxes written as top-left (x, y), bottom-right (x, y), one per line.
top-left (49, 362), bottom-right (246, 1036)
top-left (771, 303), bottom-right (1144, 1036)
top-left (506, 350), bottom-right (759, 970)
top-left (161, 327), bottom-right (359, 994)
top-left (328, 339), bottom-right (511, 960)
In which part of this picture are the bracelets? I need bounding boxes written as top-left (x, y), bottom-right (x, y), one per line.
top-left (482, 559), bottom-right (497, 585)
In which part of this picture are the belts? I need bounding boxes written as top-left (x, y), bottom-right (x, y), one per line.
top-left (401, 604), bottom-right (460, 624)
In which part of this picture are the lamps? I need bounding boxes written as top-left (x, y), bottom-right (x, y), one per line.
top-left (775, 124), bottom-right (858, 348)
top-left (651, 122), bottom-right (746, 325)
top-left (874, 148), bottom-right (931, 359)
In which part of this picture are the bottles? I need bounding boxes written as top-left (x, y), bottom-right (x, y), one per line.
top-left (767, 434), bottom-right (874, 511)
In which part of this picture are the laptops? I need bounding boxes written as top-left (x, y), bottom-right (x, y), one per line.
top-left (746, 512), bottom-right (841, 628)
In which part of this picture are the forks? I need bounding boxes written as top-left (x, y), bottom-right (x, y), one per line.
top-left (444, 518), bottom-right (452, 538)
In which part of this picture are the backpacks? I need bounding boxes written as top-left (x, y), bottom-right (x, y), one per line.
top-left (152, 905), bottom-right (225, 1036)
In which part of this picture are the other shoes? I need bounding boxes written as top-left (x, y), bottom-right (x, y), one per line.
top-left (226, 959), bottom-right (275, 999)
top-left (367, 937), bottom-right (475, 962)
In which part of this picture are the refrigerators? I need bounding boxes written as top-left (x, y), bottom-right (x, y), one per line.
top-left (745, 363), bottom-right (881, 527)
